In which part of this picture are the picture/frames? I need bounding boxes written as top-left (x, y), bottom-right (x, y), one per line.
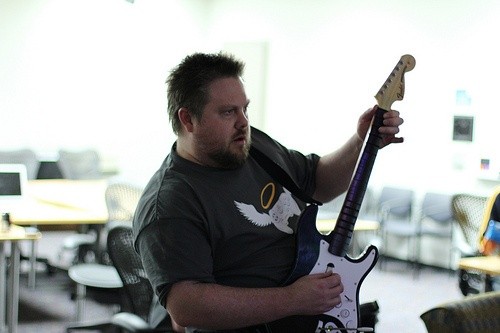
top-left (453, 115), bottom-right (474, 142)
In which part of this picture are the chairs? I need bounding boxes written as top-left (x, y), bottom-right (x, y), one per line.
top-left (107, 224), bottom-right (154, 318)
top-left (333, 185), bottom-right (455, 272)
top-left (102, 182), bottom-right (142, 221)
top-left (452, 193), bottom-right (489, 248)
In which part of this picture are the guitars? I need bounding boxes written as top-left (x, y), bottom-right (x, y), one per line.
top-left (270, 53), bottom-right (417, 333)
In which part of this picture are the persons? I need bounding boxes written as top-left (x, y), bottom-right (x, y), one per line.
top-left (130, 52), bottom-right (404, 333)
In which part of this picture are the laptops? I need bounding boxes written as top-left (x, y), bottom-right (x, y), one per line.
top-left (0, 164), bottom-right (40, 214)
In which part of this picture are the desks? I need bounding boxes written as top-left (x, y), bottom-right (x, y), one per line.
top-left (316, 216), bottom-right (380, 258)
top-left (0, 179), bottom-right (109, 333)
top-left (458, 255), bottom-right (500, 297)
